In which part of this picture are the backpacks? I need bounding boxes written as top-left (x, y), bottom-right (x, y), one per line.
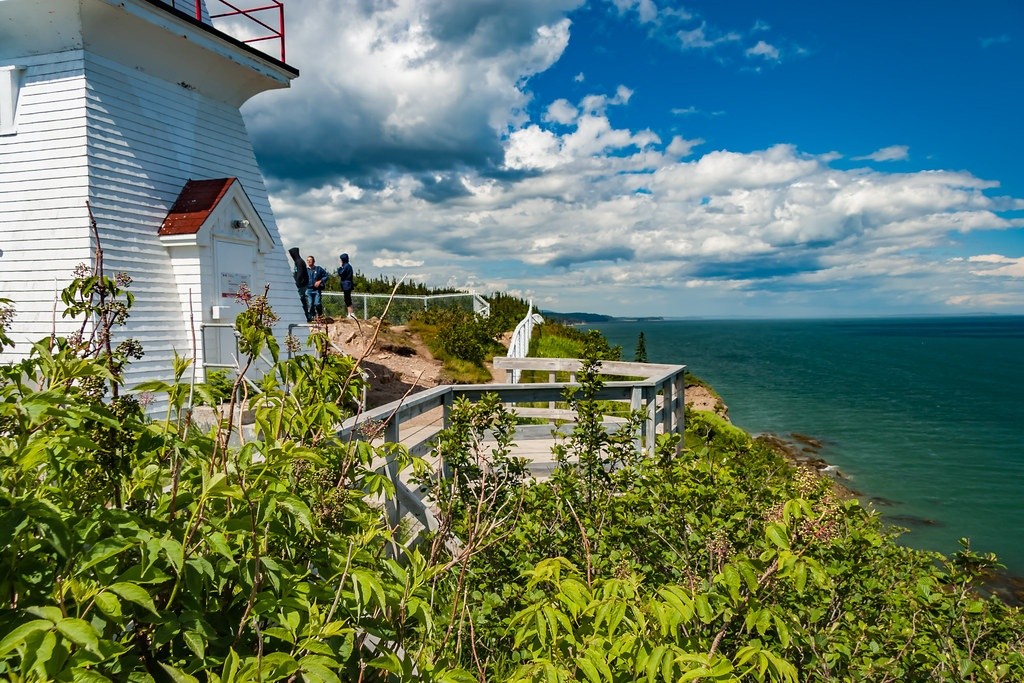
top-left (314, 266), bottom-right (325, 290)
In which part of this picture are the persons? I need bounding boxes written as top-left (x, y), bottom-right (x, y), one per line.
top-left (288, 247), bottom-right (310, 322)
top-left (304, 254), bottom-right (329, 319)
top-left (337, 253), bottom-right (357, 319)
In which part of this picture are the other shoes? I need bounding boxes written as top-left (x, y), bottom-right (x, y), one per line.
top-left (345, 313), bottom-right (358, 321)
top-left (310, 313), bottom-right (324, 320)
